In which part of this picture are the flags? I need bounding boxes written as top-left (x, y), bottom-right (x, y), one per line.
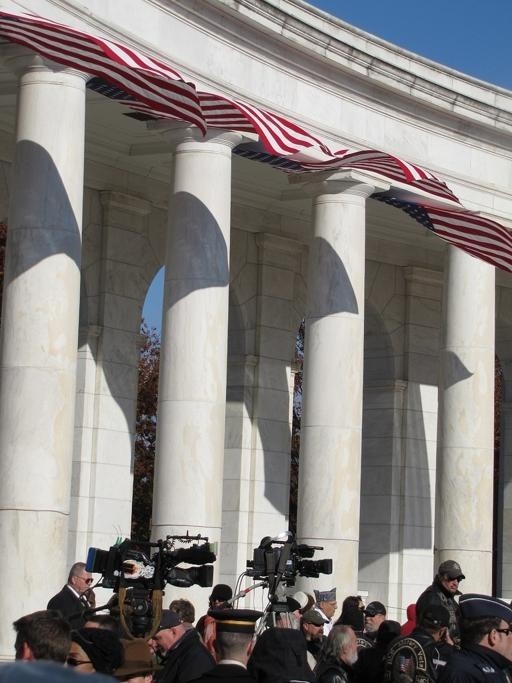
top-left (1, 11), bottom-right (511, 273)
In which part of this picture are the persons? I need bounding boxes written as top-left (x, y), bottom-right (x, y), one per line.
top-left (1, 558), bottom-right (512, 683)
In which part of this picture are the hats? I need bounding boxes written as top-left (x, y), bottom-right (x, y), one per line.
top-left (361, 601), bottom-right (386, 616)
top-left (208, 608), bottom-right (264, 633)
top-left (303, 609), bottom-right (329, 625)
top-left (438, 560), bottom-right (465, 579)
top-left (314, 586), bottom-right (336, 601)
top-left (112, 637), bottom-right (163, 676)
top-left (209, 584), bottom-right (232, 601)
top-left (459, 592), bottom-right (512, 623)
top-left (157, 608), bottom-right (181, 629)
top-left (70, 626), bottom-right (124, 673)
top-left (423, 604), bottom-right (451, 629)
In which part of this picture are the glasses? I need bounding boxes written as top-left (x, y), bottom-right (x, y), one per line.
top-left (66, 657), bottom-right (92, 665)
top-left (448, 574), bottom-right (463, 581)
top-left (76, 575), bottom-right (93, 583)
